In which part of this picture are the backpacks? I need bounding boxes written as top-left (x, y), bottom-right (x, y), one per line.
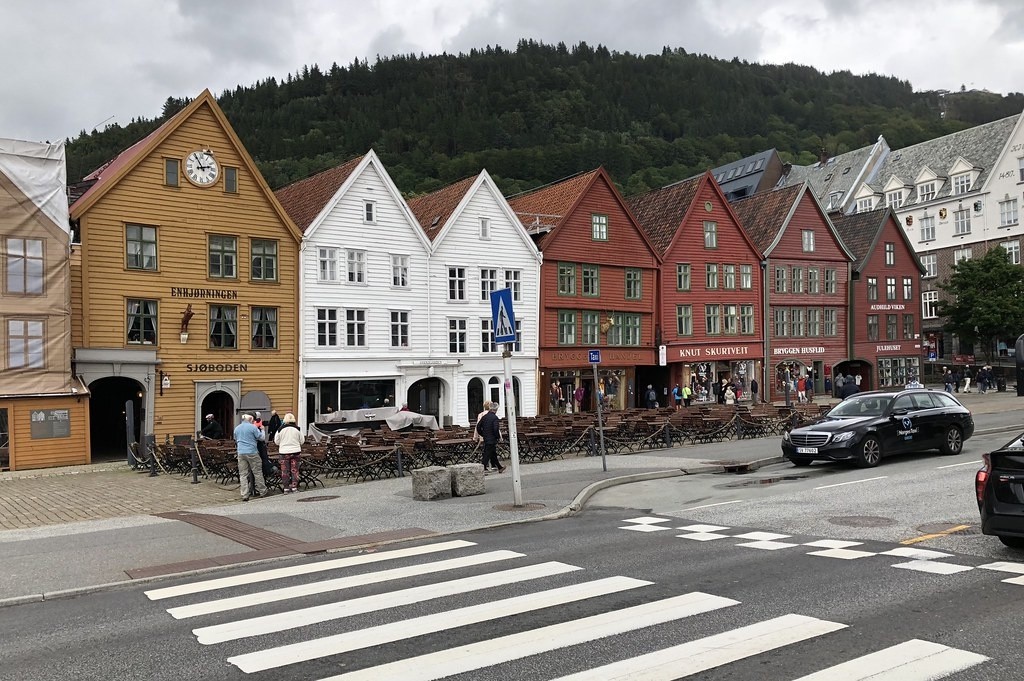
top-left (649, 390), bottom-right (656, 400)
top-left (675, 387), bottom-right (682, 396)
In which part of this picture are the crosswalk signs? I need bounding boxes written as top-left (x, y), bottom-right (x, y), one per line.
top-left (489, 287), bottom-right (518, 345)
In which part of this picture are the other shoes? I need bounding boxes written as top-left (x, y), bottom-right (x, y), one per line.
top-left (964, 391), bottom-right (967, 393)
top-left (491, 465), bottom-right (498, 469)
top-left (485, 470), bottom-right (492, 474)
top-left (968, 391), bottom-right (971, 393)
top-left (242, 495), bottom-right (249, 501)
top-left (499, 466), bottom-right (507, 473)
top-left (284, 489), bottom-right (291, 494)
top-left (261, 491), bottom-right (272, 498)
top-left (291, 488), bottom-right (300, 493)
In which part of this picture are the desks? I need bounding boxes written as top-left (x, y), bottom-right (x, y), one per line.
top-left (362, 444), bottom-right (403, 475)
top-left (409, 436), bottom-right (440, 461)
top-left (435, 437), bottom-right (473, 467)
top-left (647, 420), bottom-right (671, 447)
top-left (587, 424), bottom-right (617, 452)
top-left (268, 450), bottom-right (318, 486)
top-left (702, 415), bottom-right (723, 441)
top-left (525, 430), bottom-right (556, 459)
top-left (749, 412), bottom-right (769, 435)
top-left (338, 441), bottom-right (377, 469)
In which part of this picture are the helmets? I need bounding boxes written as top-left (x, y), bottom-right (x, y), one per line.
top-left (205, 414), bottom-right (214, 419)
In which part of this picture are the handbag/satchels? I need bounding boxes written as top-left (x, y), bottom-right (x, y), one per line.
top-left (688, 394), bottom-right (695, 400)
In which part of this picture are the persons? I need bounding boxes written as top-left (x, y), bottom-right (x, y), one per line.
top-left (942, 364), bottom-right (996, 393)
top-left (274, 414), bottom-right (304, 495)
top-left (749, 377), bottom-right (759, 406)
top-left (836, 373), bottom-right (860, 401)
top-left (361, 398), bottom-right (391, 408)
top-left (672, 384), bottom-right (681, 411)
top-left (644, 384), bottom-right (656, 408)
top-left (554, 381), bottom-right (562, 407)
top-left (268, 410), bottom-right (281, 441)
top-left (400, 402), bottom-right (409, 411)
top-left (797, 375), bottom-right (813, 403)
top-left (599, 378), bottom-right (605, 396)
top-left (606, 378), bottom-right (615, 402)
top-left (682, 384), bottom-right (691, 406)
top-left (473, 400), bottom-right (507, 473)
top-left (201, 414), bottom-right (223, 439)
top-left (695, 376), bottom-right (740, 405)
top-left (234, 412), bottom-right (268, 501)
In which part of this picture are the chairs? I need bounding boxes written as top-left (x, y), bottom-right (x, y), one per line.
top-left (129, 402), bottom-right (839, 493)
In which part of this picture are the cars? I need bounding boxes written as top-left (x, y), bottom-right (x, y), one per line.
top-left (781, 388), bottom-right (975, 469)
top-left (975, 431), bottom-right (1024, 549)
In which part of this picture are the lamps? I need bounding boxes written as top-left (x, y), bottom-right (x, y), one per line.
top-left (201, 143), bottom-right (214, 155)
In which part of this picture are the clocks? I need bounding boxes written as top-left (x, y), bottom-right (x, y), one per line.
top-left (183, 149), bottom-right (222, 188)
